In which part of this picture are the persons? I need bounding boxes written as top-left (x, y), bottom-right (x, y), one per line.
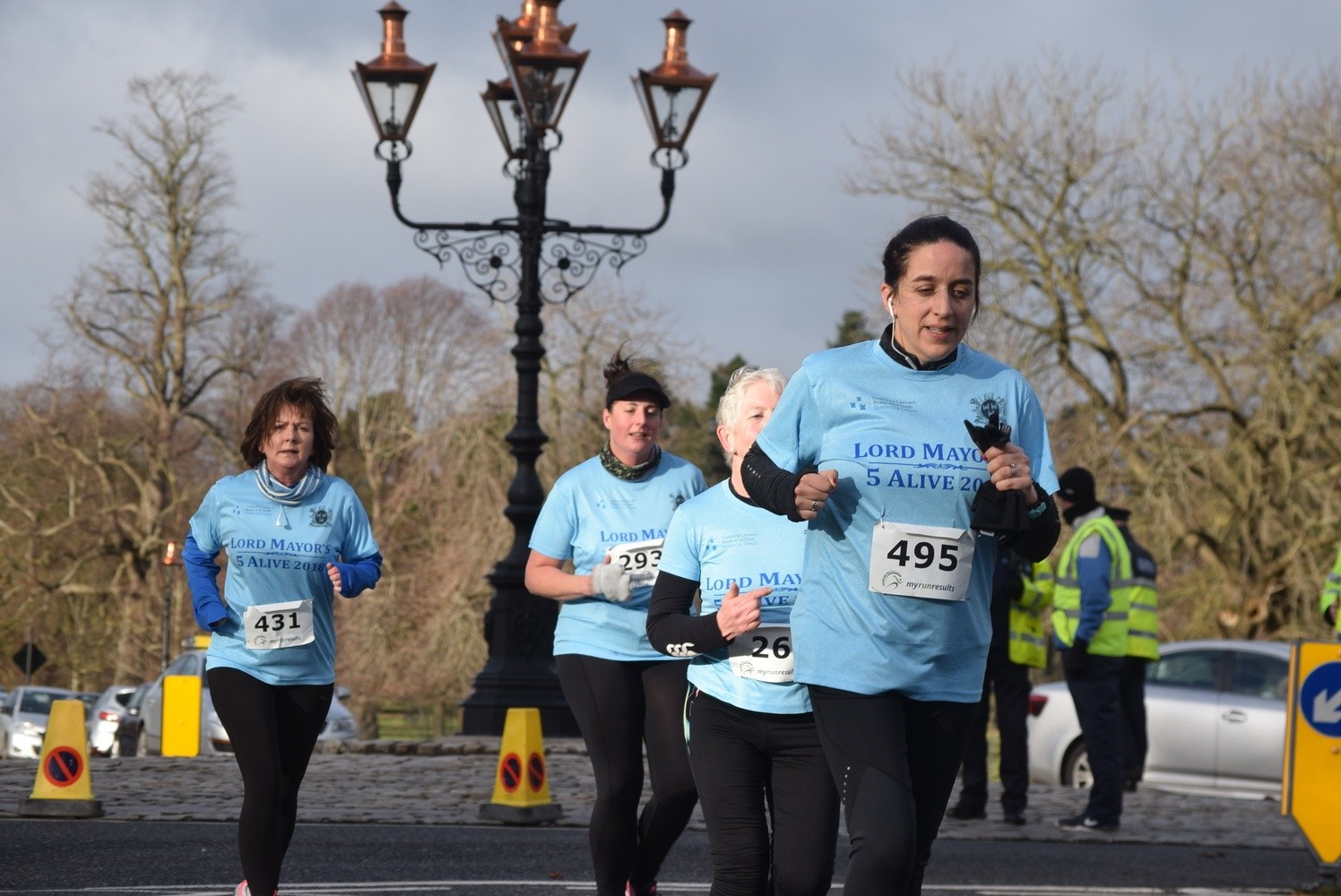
top-left (1320, 552), bottom-right (1340, 643)
top-left (525, 215), bottom-right (1061, 896)
top-left (946, 467), bottom-right (1159, 831)
top-left (182, 377), bottom-right (382, 896)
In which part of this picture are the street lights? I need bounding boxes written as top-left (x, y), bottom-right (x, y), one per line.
top-left (348, 0), bottom-right (715, 733)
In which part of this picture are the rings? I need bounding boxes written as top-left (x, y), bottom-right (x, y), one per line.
top-left (1010, 462), bottom-right (1016, 476)
top-left (811, 500), bottom-right (818, 511)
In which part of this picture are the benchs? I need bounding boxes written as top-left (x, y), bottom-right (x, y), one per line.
top-left (362, 702), bottom-right (460, 739)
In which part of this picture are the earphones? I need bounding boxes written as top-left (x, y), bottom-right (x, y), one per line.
top-left (276, 515), bottom-right (281, 526)
top-left (888, 294), bottom-right (895, 315)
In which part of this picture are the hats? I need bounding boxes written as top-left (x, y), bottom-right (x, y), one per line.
top-left (1106, 507), bottom-right (1131, 520)
top-left (606, 370), bottom-right (669, 408)
top-left (1056, 468), bottom-right (1095, 503)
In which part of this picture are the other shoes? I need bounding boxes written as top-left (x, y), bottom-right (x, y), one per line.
top-left (1005, 810), bottom-right (1027, 826)
top-left (1124, 782), bottom-right (1137, 793)
top-left (1057, 813), bottom-right (1120, 832)
top-left (234, 880), bottom-right (278, 896)
top-left (947, 802), bottom-right (989, 820)
top-left (625, 882), bottom-right (657, 896)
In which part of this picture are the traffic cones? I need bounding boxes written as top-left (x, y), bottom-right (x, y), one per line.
top-left (28, 699), bottom-right (96, 801)
top-left (477, 707), bottom-right (564, 827)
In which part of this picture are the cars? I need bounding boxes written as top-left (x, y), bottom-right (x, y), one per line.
top-left (0, 684), bottom-right (84, 760)
top-left (83, 683), bottom-right (153, 758)
top-left (134, 635), bottom-right (360, 758)
top-left (1025, 639), bottom-right (1292, 804)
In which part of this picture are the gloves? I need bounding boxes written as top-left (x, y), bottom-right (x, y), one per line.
top-left (1072, 639), bottom-right (1089, 660)
